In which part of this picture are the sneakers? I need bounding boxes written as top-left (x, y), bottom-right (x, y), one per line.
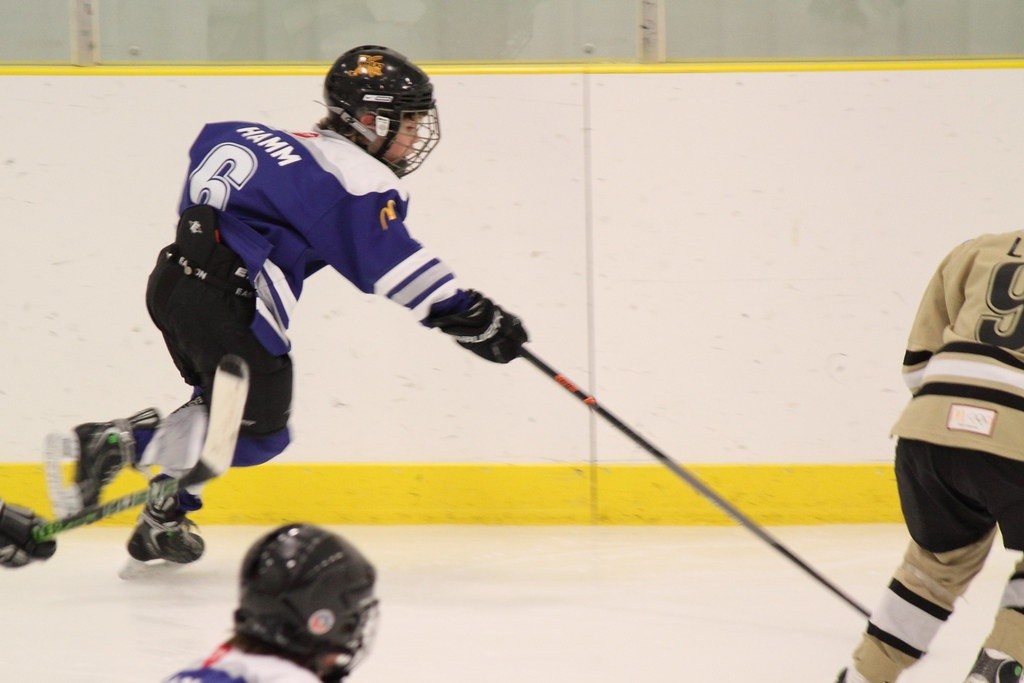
top-left (115, 507), bottom-right (207, 581)
top-left (45, 417), bottom-right (125, 521)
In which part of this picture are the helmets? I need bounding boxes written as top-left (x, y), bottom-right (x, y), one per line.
top-left (233, 522), bottom-right (378, 680)
top-left (323, 43), bottom-right (441, 174)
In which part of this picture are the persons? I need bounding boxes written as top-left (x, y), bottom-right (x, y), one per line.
top-left (45, 41), bottom-right (527, 563)
top-left (833, 229), bottom-right (1024, 682)
top-left (163, 521), bottom-right (379, 682)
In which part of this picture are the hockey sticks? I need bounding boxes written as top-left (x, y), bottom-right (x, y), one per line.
top-left (515, 343), bottom-right (877, 621)
top-left (31, 355), bottom-right (252, 544)
